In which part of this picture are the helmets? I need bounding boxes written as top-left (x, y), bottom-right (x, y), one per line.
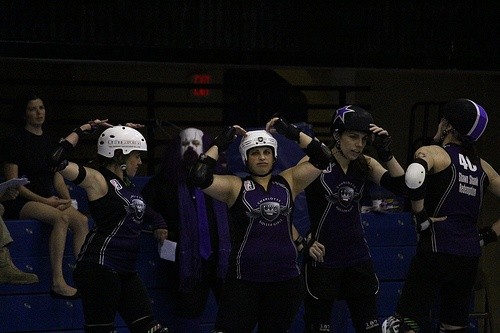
top-left (97, 125), bottom-right (147, 158)
top-left (329, 105), bottom-right (375, 136)
top-left (444, 97), bottom-right (488, 141)
top-left (238, 129), bottom-right (278, 166)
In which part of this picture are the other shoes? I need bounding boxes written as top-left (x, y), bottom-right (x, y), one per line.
top-left (50, 285), bottom-right (81, 300)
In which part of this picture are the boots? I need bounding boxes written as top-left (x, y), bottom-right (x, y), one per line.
top-left (0, 248), bottom-right (39, 284)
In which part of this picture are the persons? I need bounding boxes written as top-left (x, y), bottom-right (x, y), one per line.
top-left (52, 116), bottom-right (156, 333)
top-left (141, 202), bottom-right (168, 246)
top-left (155, 127), bottom-right (236, 333)
top-left (285, 104), bottom-right (409, 333)
top-left (0, 187), bottom-right (40, 283)
top-left (4, 95), bottom-right (94, 296)
top-left (404, 98), bottom-right (500, 333)
top-left (192, 117), bottom-right (332, 332)
